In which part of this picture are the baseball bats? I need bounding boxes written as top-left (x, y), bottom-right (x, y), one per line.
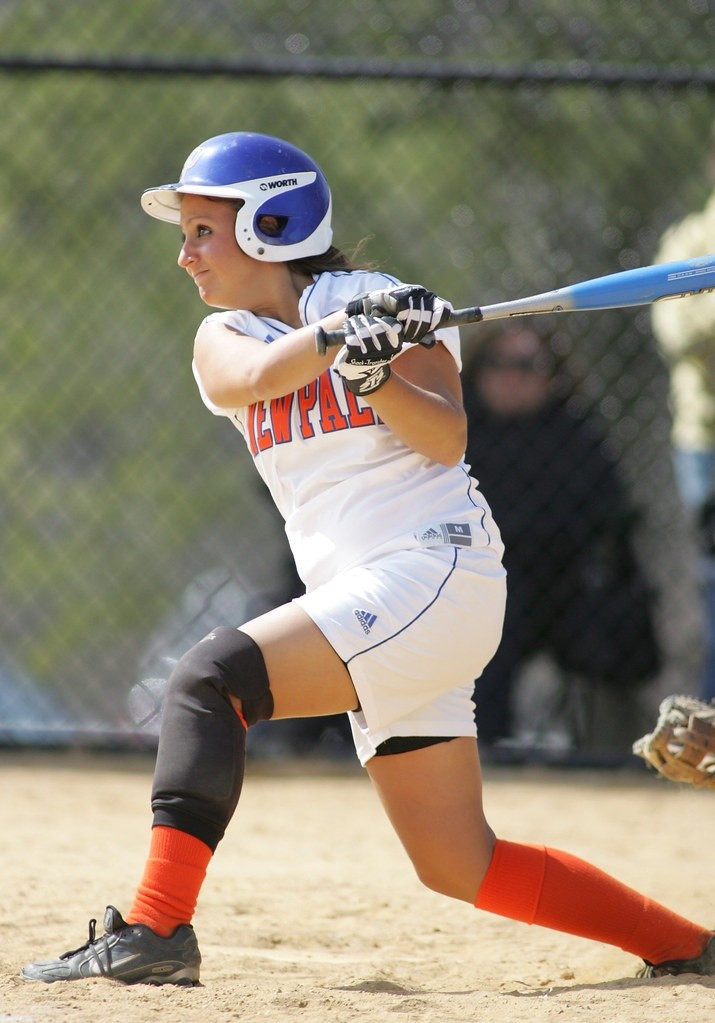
top-left (315, 253), bottom-right (715, 356)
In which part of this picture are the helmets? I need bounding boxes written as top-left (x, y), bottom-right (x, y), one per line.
top-left (140, 131), bottom-right (335, 262)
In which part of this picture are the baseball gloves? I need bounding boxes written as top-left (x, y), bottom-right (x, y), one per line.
top-left (633, 694), bottom-right (715, 785)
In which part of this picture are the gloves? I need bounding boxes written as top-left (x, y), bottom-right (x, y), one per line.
top-left (346, 285), bottom-right (455, 349)
top-left (337, 304), bottom-right (404, 396)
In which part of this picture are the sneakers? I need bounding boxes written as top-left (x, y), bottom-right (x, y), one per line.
top-left (20, 905), bottom-right (201, 987)
top-left (635, 930), bottom-right (715, 979)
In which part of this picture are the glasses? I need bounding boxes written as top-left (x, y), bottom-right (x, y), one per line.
top-left (480, 352), bottom-right (534, 373)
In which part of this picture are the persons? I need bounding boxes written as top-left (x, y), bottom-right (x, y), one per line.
top-left (22, 130), bottom-right (715, 986)
